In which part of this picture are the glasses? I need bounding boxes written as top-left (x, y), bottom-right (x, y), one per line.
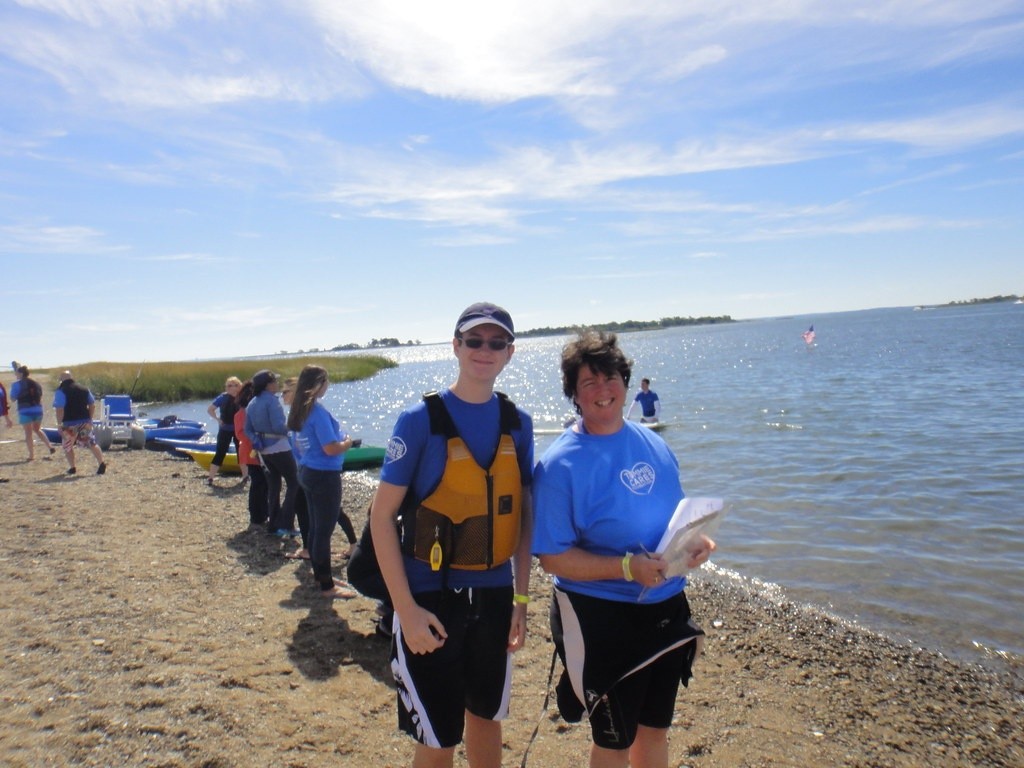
top-left (455, 335), bottom-right (512, 350)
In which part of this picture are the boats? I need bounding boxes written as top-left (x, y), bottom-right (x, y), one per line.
top-left (534, 422), bottom-right (667, 437)
top-left (39, 417), bottom-right (204, 447)
top-left (156, 435), bottom-right (238, 456)
top-left (177, 444), bottom-right (390, 474)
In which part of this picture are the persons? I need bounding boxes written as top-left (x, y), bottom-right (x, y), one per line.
top-left (0, 381), bottom-right (13, 428)
top-left (9, 364), bottom-right (56, 462)
top-left (284, 363), bottom-right (358, 597)
top-left (51, 370), bottom-right (107, 475)
top-left (626, 378), bottom-right (662, 422)
top-left (234, 365), bottom-right (360, 559)
top-left (621, 550), bottom-right (634, 582)
top-left (204, 376), bottom-right (250, 485)
top-left (533, 324), bottom-right (726, 768)
top-left (371, 302), bottom-right (533, 768)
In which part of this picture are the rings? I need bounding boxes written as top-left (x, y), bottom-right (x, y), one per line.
top-left (655, 577), bottom-right (658, 583)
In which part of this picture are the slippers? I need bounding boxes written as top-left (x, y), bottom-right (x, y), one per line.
top-left (284, 548), bottom-right (311, 560)
top-left (314, 576), bottom-right (349, 588)
top-left (319, 586), bottom-right (358, 599)
top-left (335, 550), bottom-right (351, 559)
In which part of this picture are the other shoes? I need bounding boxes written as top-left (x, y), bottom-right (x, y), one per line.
top-left (207, 478), bottom-right (213, 485)
top-left (97, 463), bottom-right (106, 474)
top-left (241, 476), bottom-right (249, 484)
top-left (276, 527), bottom-right (300, 536)
top-left (66, 466), bottom-right (76, 473)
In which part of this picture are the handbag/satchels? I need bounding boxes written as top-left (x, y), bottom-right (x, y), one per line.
top-left (347, 391), bottom-right (443, 601)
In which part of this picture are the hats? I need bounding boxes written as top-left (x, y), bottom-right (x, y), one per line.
top-left (454, 302), bottom-right (515, 341)
top-left (252, 370), bottom-right (280, 385)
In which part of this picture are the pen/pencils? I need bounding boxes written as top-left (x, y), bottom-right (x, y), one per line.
top-left (638, 543), bottom-right (667, 580)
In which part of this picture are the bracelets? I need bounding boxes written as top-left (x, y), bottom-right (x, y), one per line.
top-left (512, 593), bottom-right (532, 603)
